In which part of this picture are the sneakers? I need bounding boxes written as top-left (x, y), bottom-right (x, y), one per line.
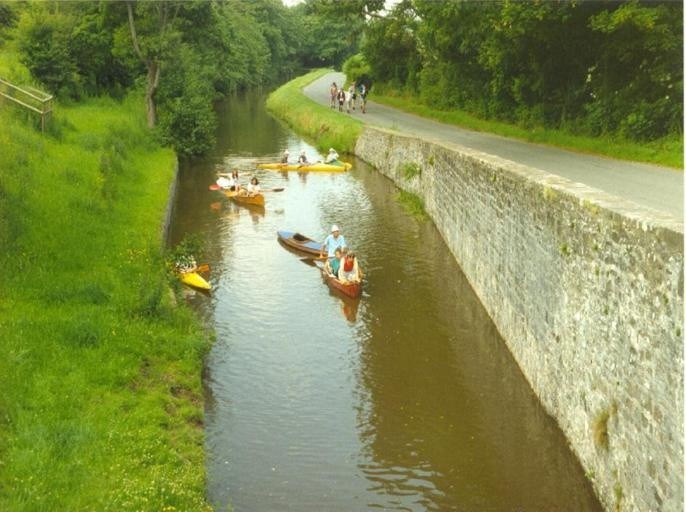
top-left (330, 105), bottom-right (365, 113)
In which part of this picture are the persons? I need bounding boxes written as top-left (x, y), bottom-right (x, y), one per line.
top-left (328, 248), bottom-right (344, 277)
top-left (280, 150), bottom-right (289, 165)
top-left (321, 225), bottom-right (346, 263)
top-left (325, 148), bottom-right (340, 163)
top-left (247, 178), bottom-right (260, 193)
top-left (329, 80), bottom-right (368, 114)
top-left (336, 251), bottom-right (359, 282)
top-left (175, 255), bottom-right (198, 273)
top-left (229, 170), bottom-right (241, 192)
top-left (298, 150), bottom-right (307, 165)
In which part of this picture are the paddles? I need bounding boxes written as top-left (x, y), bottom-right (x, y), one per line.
top-left (258, 188), bottom-right (285, 192)
top-left (191, 264), bottom-right (211, 272)
top-left (208, 184), bottom-right (234, 191)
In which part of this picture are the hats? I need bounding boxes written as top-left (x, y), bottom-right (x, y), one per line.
top-left (332, 225), bottom-right (339, 233)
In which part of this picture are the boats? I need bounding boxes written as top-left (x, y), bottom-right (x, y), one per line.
top-left (277, 230), bottom-right (329, 256)
top-left (217, 176), bottom-right (265, 206)
top-left (176, 265), bottom-right (211, 291)
top-left (325, 258), bottom-right (363, 299)
top-left (256, 162), bottom-right (352, 172)
